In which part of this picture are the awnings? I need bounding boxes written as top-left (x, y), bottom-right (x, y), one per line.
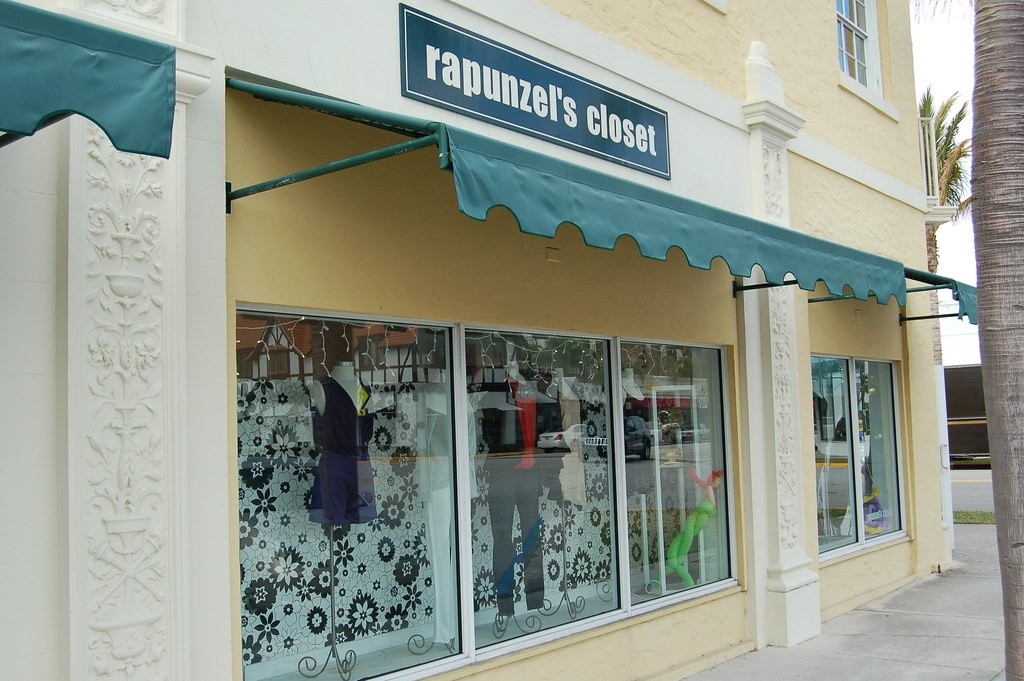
top-left (224, 78), bottom-right (907, 309)
top-left (0, 0), bottom-right (177, 161)
top-left (809, 266), bottom-right (978, 324)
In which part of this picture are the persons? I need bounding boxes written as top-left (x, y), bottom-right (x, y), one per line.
top-left (623, 367), bottom-right (651, 459)
top-left (309, 361), bottom-right (380, 524)
top-left (479, 368), bottom-right (565, 616)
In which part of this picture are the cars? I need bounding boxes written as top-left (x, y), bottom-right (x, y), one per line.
top-left (671, 422), bottom-right (711, 444)
top-left (537, 415), bottom-right (651, 458)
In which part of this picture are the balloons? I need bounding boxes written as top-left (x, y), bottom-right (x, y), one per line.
top-left (649, 466), bottom-right (723, 591)
